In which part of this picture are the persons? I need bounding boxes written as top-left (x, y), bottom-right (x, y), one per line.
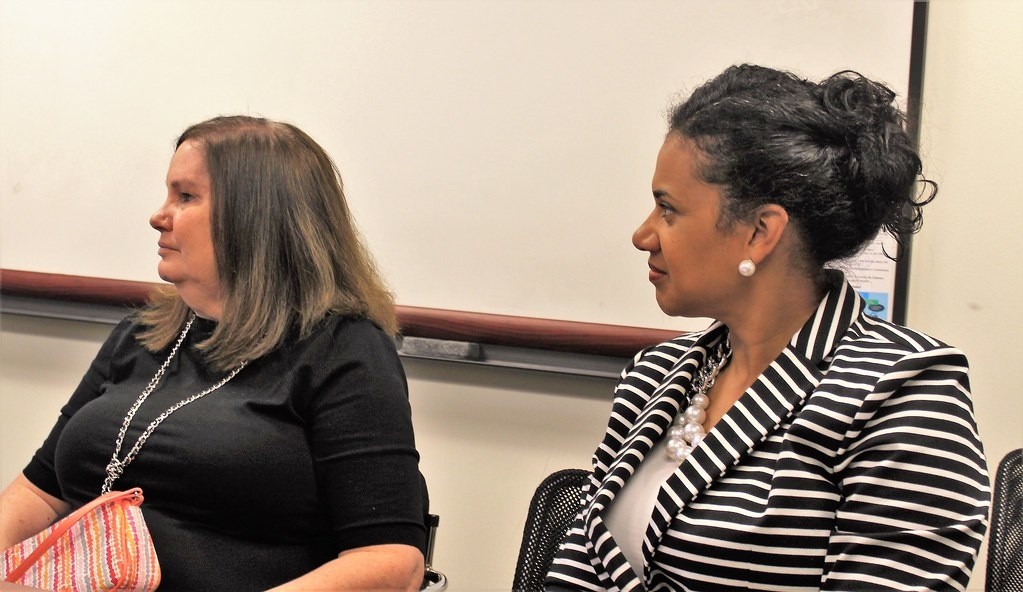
top-left (0, 116), bottom-right (430, 592)
top-left (543, 64), bottom-right (990, 592)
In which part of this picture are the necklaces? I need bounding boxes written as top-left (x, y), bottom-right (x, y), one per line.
top-left (665, 338), bottom-right (732, 465)
top-left (102, 313), bottom-right (249, 494)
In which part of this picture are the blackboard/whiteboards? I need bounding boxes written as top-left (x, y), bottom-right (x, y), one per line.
top-left (0, 0), bottom-right (933, 387)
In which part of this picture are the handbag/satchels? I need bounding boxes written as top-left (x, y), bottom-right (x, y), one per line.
top-left (0, 487), bottom-right (160, 592)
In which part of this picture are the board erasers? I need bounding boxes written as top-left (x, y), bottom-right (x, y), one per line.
top-left (400, 336), bottom-right (481, 362)
